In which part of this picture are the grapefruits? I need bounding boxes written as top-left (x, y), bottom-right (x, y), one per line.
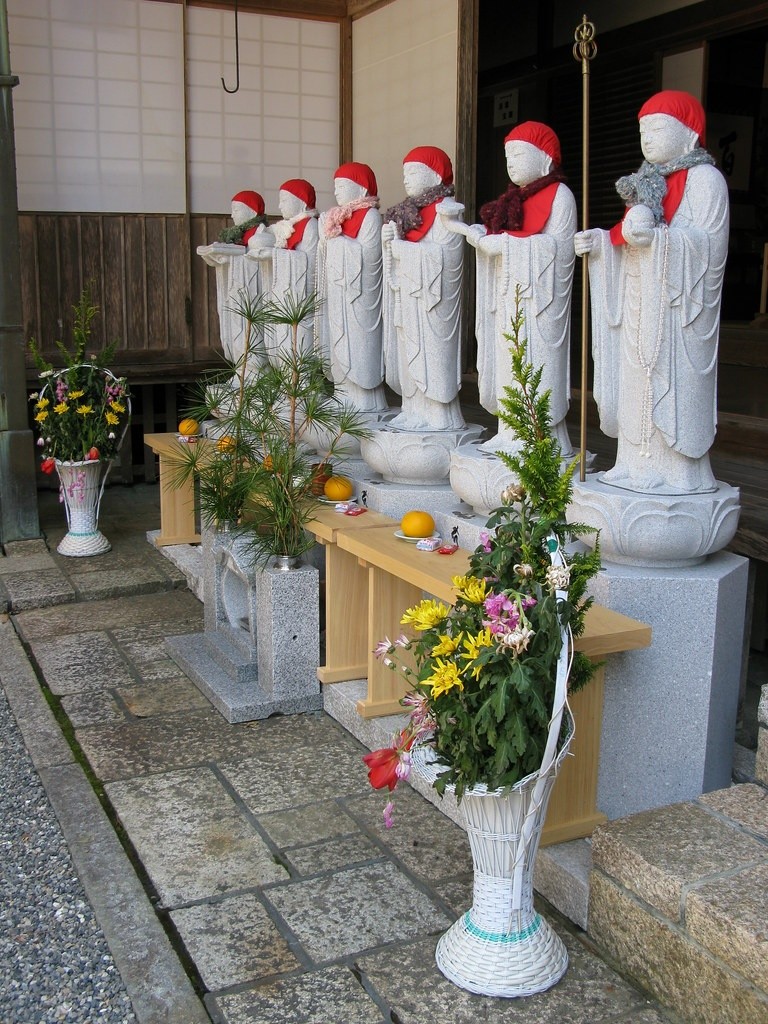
top-left (324, 476), bottom-right (353, 501)
top-left (401, 510), bottom-right (434, 538)
top-left (216, 434), bottom-right (236, 455)
top-left (264, 454), bottom-right (280, 471)
top-left (179, 420), bottom-right (198, 434)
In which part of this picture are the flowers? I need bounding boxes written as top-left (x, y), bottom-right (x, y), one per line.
top-left (361, 279), bottom-right (610, 831)
top-left (25, 276), bottom-right (134, 507)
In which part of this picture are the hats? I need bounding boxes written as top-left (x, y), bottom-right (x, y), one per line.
top-left (280, 178), bottom-right (316, 209)
top-left (403, 146), bottom-right (454, 186)
top-left (638, 90), bottom-right (704, 145)
top-left (504, 121), bottom-right (561, 168)
top-left (334, 162), bottom-right (377, 197)
top-left (232, 191), bottom-right (265, 216)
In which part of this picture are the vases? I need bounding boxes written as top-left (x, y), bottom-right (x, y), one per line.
top-left (408, 691), bottom-right (568, 997)
top-left (42, 451), bottom-right (114, 559)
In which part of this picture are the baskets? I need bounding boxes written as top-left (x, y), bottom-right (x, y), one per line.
top-left (41, 364), bottom-right (132, 557)
top-left (409, 529), bottom-right (569, 998)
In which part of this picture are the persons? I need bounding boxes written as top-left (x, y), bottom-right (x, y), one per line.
top-left (463, 121), bottom-right (578, 456)
top-left (196, 192), bottom-right (274, 391)
top-left (313, 159), bottom-right (390, 409)
top-left (574, 90), bottom-right (731, 494)
top-left (244, 177), bottom-right (327, 403)
top-left (376, 141), bottom-right (467, 431)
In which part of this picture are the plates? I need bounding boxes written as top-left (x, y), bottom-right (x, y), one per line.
top-left (394, 530), bottom-right (440, 544)
top-left (292, 476), bottom-right (310, 488)
top-left (214, 451), bottom-right (235, 460)
top-left (175, 432), bottom-right (203, 437)
top-left (317, 494), bottom-right (358, 506)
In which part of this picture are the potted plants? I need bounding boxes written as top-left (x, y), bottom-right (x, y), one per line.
top-left (153, 280), bottom-right (379, 571)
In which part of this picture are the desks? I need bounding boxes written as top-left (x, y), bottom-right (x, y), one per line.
top-left (217, 471), bottom-right (403, 683)
top-left (142, 430), bottom-right (245, 546)
top-left (337, 523), bottom-right (654, 848)
